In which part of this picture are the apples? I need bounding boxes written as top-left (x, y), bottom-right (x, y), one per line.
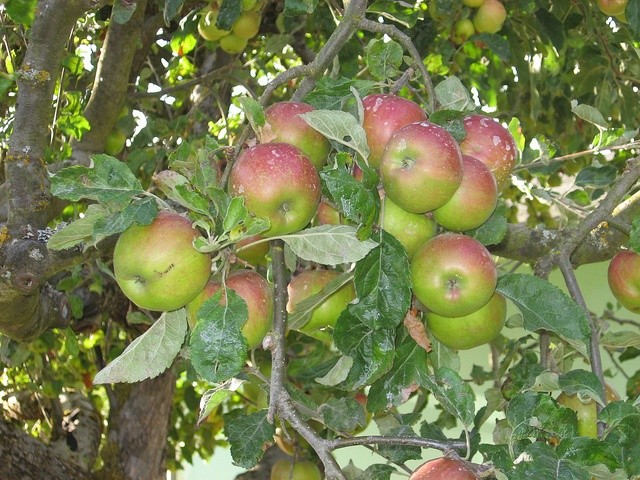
top-left (347, 396), bottom-right (373, 437)
top-left (273, 419), bottom-right (312, 454)
top-left (433, 154), bottom-right (498, 234)
top-left (185, 266), bottom-right (276, 352)
top-left (103, 125), bottom-right (126, 157)
top-left (608, 249), bottom-right (639, 314)
top-left (473, 0), bottom-right (508, 34)
top-left (446, 113), bottom-right (521, 193)
top-left (287, 268), bottom-right (358, 343)
top-left (614, 9), bottom-right (629, 25)
top-left (311, 199), bottom-right (361, 231)
top-left (112, 212), bottom-right (212, 313)
top-left (355, 93), bottom-right (429, 172)
top-left (226, 142), bottom-right (321, 238)
top-left (380, 120), bottom-right (463, 215)
top-left (460, 0), bottom-right (486, 9)
top-left (197, 0), bottom-right (264, 55)
top-left (374, 195), bottom-right (439, 264)
top-left (245, 353), bottom-right (278, 385)
top-left (263, 100), bottom-right (334, 172)
top-left (269, 458), bottom-right (323, 480)
top-left (451, 18), bottom-right (474, 45)
top-left (423, 290), bottom-right (507, 351)
top-left (596, 0), bottom-right (630, 17)
top-left (556, 380), bottom-right (622, 441)
top-left (226, 236), bottom-right (271, 269)
top-left (409, 232), bottom-right (499, 318)
top-left (408, 457), bottom-right (479, 480)
top-left (426, 1), bottom-right (443, 22)
